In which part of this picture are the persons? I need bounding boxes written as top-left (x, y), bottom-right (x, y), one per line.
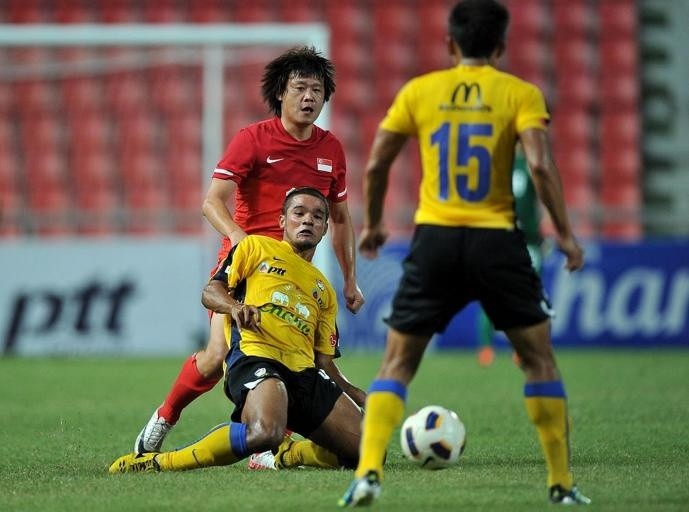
top-left (107, 187), bottom-right (387, 475)
top-left (133, 45), bottom-right (366, 470)
top-left (337, 1), bottom-right (595, 508)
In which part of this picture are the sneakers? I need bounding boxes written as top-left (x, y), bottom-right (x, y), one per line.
top-left (249, 430), bottom-right (297, 471)
top-left (109, 400), bottom-right (175, 474)
top-left (338, 470), bottom-right (379, 507)
top-left (550, 484), bottom-right (591, 505)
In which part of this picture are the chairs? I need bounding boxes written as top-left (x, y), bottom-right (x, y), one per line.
top-left (0, 1), bottom-right (648, 241)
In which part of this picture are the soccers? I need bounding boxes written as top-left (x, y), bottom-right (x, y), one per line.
top-left (400, 405), bottom-right (467, 469)
